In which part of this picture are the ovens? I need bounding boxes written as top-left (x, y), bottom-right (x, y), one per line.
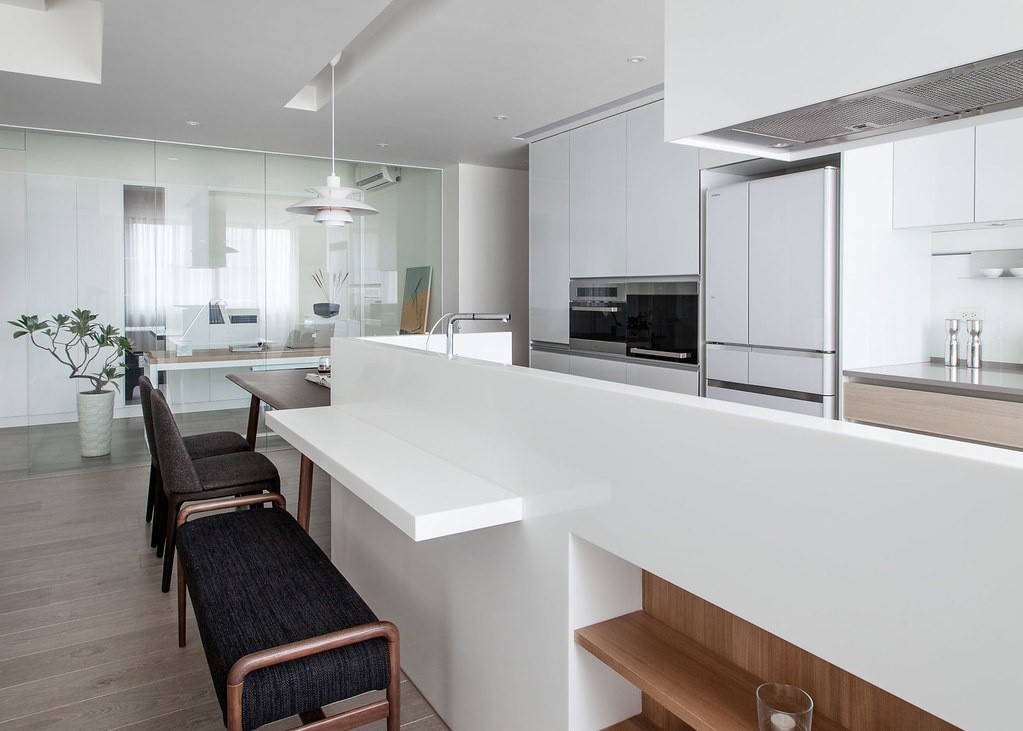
top-left (568, 282), bottom-right (626, 357)
top-left (626, 283), bottom-right (698, 365)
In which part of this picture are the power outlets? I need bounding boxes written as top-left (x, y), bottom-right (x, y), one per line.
top-left (955, 306), bottom-right (984, 323)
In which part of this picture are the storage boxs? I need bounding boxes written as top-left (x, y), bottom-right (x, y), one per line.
top-left (176, 340), bottom-right (193, 357)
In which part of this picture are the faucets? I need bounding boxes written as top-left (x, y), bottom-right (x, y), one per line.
top-left (445, 312), bottom-right (511, 356)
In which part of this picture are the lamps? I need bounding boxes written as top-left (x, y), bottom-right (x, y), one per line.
top-left (284, 50), bottom-right (382, 228)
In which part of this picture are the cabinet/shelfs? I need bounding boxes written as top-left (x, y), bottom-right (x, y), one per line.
top-left (892, 106), bottom-right (1023, 233)
top-left (573, 566), bottom-right (968, 731)
top-left (527, 345), bottom-right (706, 397)
top-left (528, 131), bottom-right (569, 347)
top-left (570, 98), bottom-right (702, 284)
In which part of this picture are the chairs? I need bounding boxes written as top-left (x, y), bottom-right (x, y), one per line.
top-left (124, 338), bottom-right (164, 401)
top-left (150, 388), bottom-right (282, 594)
top-left (138, 374), bottom-right (256, 549)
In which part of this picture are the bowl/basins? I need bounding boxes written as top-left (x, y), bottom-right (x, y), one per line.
top-left (980, 268), bottom-right (1004, 277)
top-left (1010, 268), bottom-right (1023, 276)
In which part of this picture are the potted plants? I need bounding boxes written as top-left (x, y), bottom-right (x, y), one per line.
top-left (6, 308), bottom-right (137, 459)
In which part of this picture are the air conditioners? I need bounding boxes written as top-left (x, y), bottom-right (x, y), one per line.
top-left (354, 162), bottom-right (401, 192)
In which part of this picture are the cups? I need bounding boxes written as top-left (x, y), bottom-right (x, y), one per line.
top-left (316, 357), bottom-right (331, 377)
top-left (756, 682), bottom-right (813, 731)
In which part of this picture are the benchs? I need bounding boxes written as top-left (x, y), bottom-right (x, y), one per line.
top-left (173, 492), bottom-right (402, 731)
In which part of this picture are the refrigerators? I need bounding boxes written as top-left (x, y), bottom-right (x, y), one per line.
top-left (704, 167), bottom-right (838, 422)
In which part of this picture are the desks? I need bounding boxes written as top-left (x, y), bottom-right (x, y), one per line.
top-left (263, 405), bottom-right (523, 543)
top-left (142, 347), bottom-right (331, 390)
top-left (225, 367), bottom-right (331, 535)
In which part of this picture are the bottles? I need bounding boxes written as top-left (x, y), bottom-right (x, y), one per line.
top-left (944, 318), bottom-right (961, 367)
top-left (965, 319), bottom-right (982, 369)
